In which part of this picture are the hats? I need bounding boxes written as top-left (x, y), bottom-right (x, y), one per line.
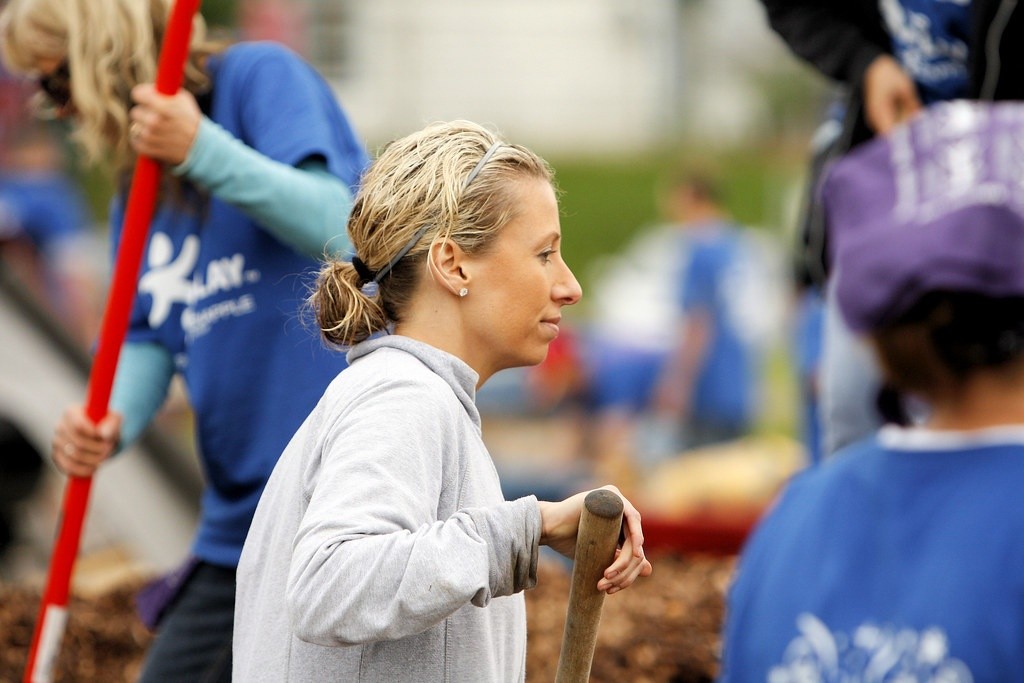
top-left (816, 100), bottom-right (1024, 328)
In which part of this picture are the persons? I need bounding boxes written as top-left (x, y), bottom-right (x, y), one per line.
top-left (230, 119), bottom-right (651, 681)
top-left (0, 0), bottom-right (396, 682)
top-left (477, 85), bottom-right (865, 518)
top-left (715, 99), bottom-right (1024, 681)
top-left (760, 0), bottom-right (1022, 469)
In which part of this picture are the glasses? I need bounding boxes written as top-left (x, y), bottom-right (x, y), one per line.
top-left (40, 68), bottom-right (84, 105)
